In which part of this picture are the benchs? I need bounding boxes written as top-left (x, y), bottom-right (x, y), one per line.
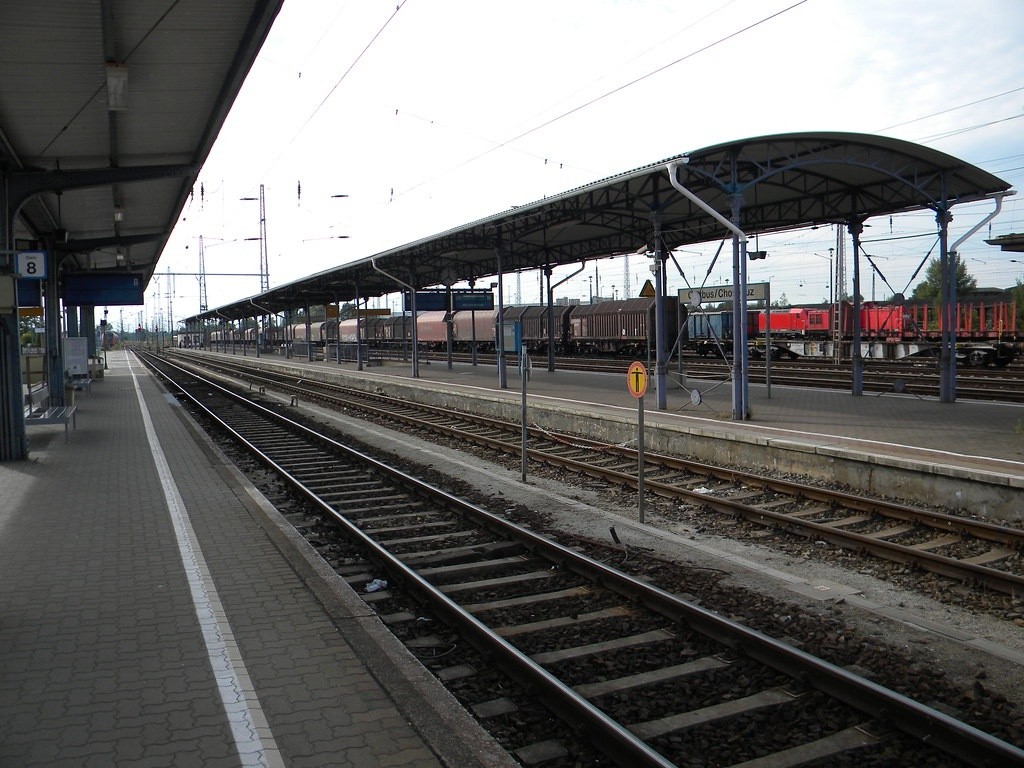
top-left (64, 368), bottom-right (91, 398)
top-left (23, 386), bottom-right (79, 445)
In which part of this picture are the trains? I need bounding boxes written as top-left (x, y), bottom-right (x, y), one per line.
top-left (100, 330), bottom-right (119, 346)
top-left (172, 288), bottom-right (1022, 369)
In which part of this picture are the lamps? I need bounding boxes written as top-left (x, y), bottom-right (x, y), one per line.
top-left (106, 64), bottom-right (129, 111)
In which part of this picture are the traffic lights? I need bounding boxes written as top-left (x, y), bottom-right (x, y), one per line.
top-left (138, 326), bottom-right (141, 332)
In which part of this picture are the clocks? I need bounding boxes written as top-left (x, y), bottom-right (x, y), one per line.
top-left (439, 267), bottom-right (458, 286)
